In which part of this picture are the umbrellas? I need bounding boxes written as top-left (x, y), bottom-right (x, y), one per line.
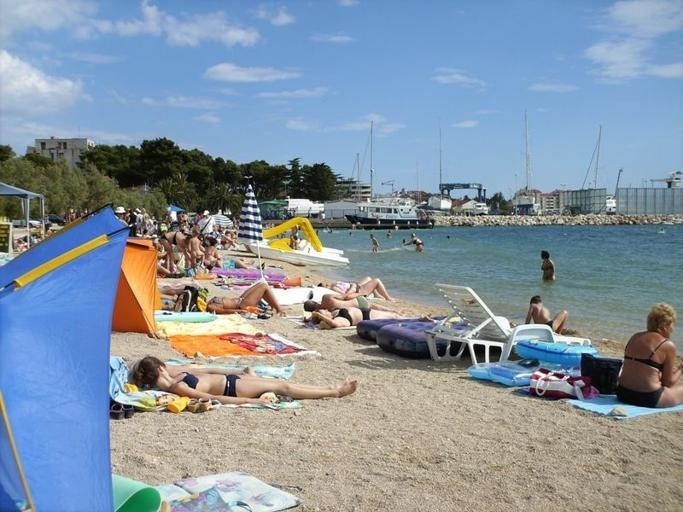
top-left (236, 183), bottom-right (264, 275)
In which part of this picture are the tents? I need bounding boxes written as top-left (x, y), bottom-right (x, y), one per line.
top-left (0, 206), bottom-right (135, 511)
top-left (110, 237), bottom-right (160, 338)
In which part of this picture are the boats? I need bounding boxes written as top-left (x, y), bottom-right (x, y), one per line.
top-left (344, 204), bottom-right (434, 230)
top-left (243, 238), bottom-right (350, 267)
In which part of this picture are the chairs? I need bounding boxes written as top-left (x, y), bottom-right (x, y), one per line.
top-left (424, 283), bottom-right (591, 368)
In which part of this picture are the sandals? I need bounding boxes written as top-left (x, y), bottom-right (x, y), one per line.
top-left (186, 398), bottom-right (220, 413)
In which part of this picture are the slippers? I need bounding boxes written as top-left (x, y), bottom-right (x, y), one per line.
top-left (121, 403), bottom-right (134, 419)
top-left (109, 402), bottom-right (123, 419)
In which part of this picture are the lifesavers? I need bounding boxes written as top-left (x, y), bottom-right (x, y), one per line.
top-left (515, 339), bottom-right (598, 367)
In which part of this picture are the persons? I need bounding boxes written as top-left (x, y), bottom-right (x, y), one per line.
top-left (541, 250), bottom-right (555, 281)
top-left (524, 295), bottom-right (568, 334)
top-left (616, 302), bottom-right (682, 407)
top-left (265, 207), bottom-right (427, 255)
top-left (160, 281), bottom-right (286, 317)
top-left (15, 207), bottom-right (249, 278)
top-left (134, 356), bottom-right (357, 406)
top-left (305, 275), bottom-right (408, 329)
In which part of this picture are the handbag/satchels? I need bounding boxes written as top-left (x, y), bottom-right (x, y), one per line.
top-left (580, 351), bottom-right (622, 395)
top-left (530, 367), bottom-right (591, 400)
